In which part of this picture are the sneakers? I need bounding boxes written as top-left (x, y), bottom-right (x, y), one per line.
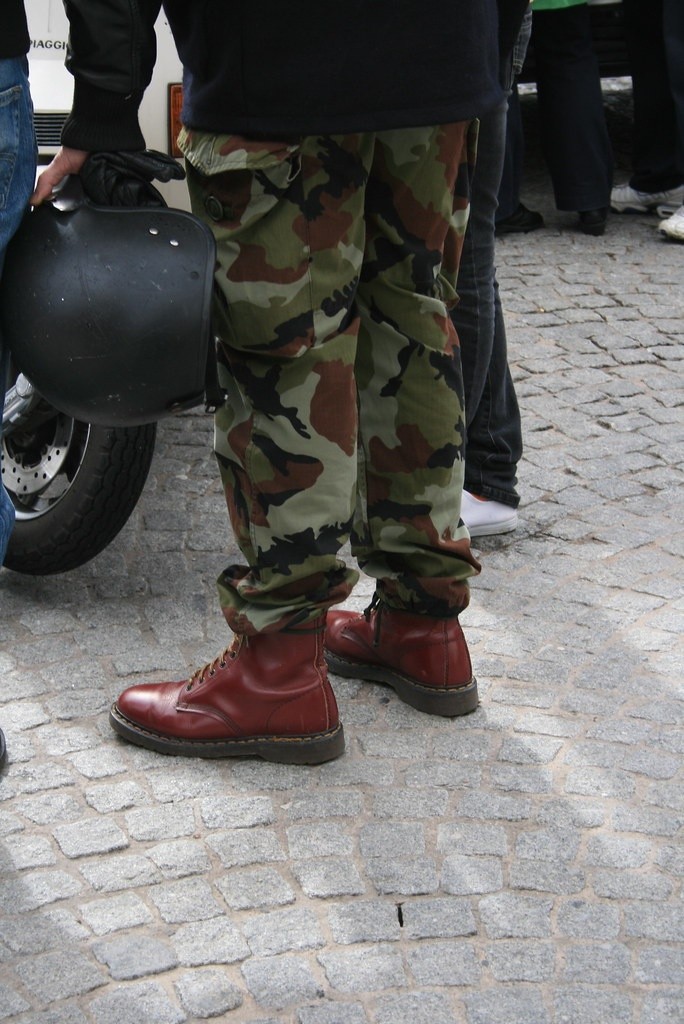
top-left (609, 181), bottom-right (684, 212)
top-left (658, 205), bottom-right (684, 240)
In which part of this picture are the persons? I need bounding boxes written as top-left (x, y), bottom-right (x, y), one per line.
top-left (438, 0), bottom-right (537, 543)
top-left (29, 0), bottom-right (487, 769)
top-left (0, 1), bottom-right (43, 257)
top-left (495, 0), bottom-right (684, 244)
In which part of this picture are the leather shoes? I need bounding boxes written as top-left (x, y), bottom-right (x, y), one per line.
top-left (107, 611), bottom-right (344, 765)
top-left (320, 609), bottom-right (479, 717)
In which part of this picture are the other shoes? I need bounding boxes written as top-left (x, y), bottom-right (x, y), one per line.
top-left (496, 201), bottom-right (544, 232)
top-left (457, 489), bottom-right (518, 538)
top-left (579, 209), bottom-right (606, 234)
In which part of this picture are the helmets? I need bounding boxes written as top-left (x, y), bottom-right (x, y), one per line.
top-left (1, 178), bottom-right (230, 429)
